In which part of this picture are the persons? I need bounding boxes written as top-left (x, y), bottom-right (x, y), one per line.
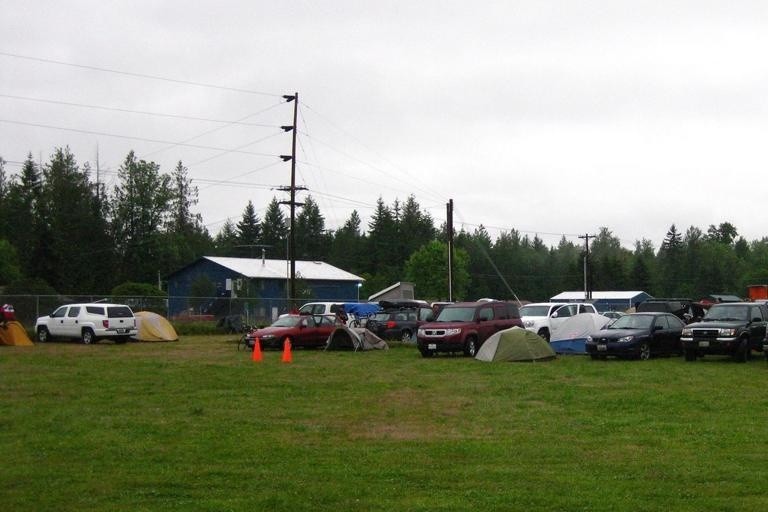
top-left (335, 305), bottom-right (349, 325)
top-left (426, 303), bottom-right (440, 322)
top-left (0, 299), bottom-right (18, 323)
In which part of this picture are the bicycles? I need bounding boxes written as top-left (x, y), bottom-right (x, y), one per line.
top-left (237, 326), bottom-right (258, 352)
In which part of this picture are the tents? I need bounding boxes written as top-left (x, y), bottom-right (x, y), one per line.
top-left (128, 309), bottom-right (179, 341)
top-left (474, 324), bottom-right (557, 363)
top-left (0, 318), bottom-right (36, 347)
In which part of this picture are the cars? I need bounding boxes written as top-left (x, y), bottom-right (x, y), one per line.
top-left (33, 302), bottom-right (139, 345)
